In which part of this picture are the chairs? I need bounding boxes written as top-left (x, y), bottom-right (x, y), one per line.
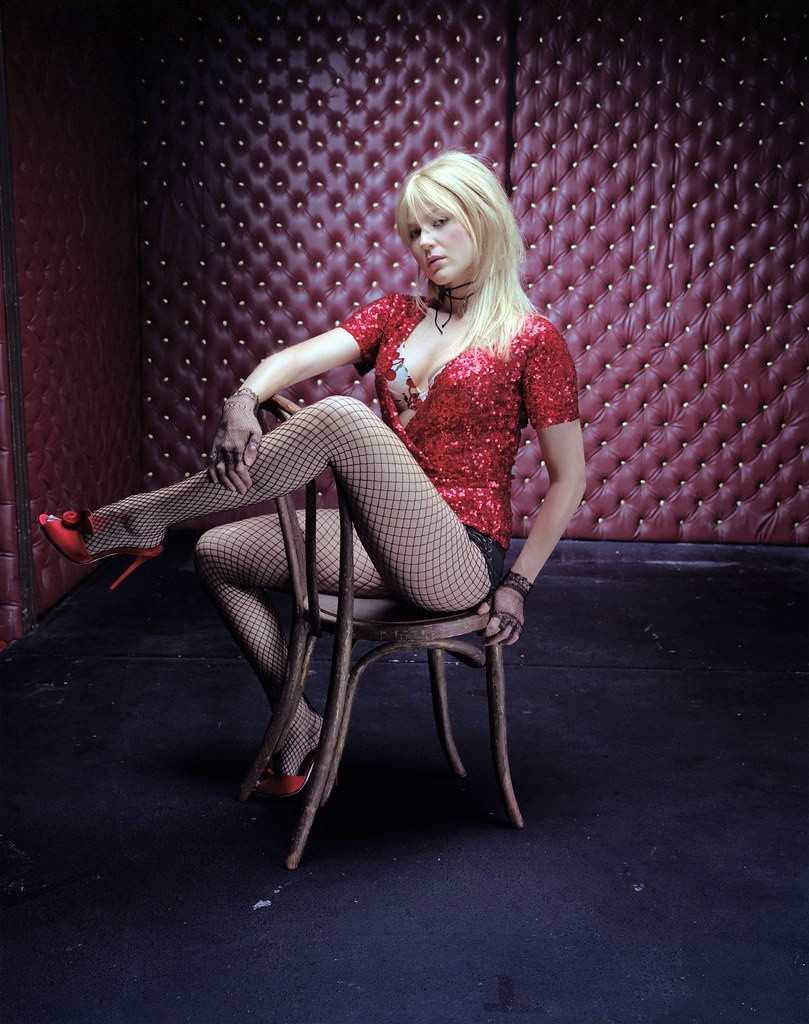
top-left (226, 376), bottom-right (528, 873)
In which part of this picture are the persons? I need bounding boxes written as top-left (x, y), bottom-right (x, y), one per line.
top-left (32, 148), bottom-right (587, 803)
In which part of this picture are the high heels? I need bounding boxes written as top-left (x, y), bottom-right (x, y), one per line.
top-left (252, 748), bottom-right (319, 799)
top-left (38, 507), bottom-right (162, 593)
